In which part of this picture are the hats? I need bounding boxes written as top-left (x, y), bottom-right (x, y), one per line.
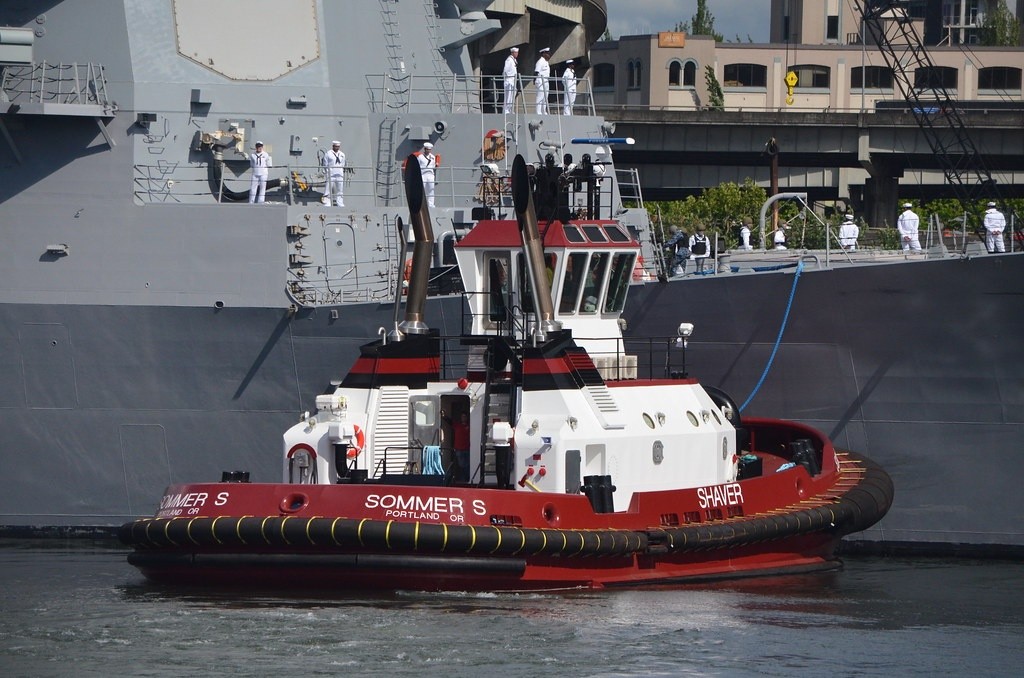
top-left (423, 142), bottom-right (433, 149)
top-left (987, 202), bottom-right (996, 206)
top-left (566, 60), bottom-right (574, 65)
top-left (845, 214), bottom-right (854, 219)
top-left (332, 141), bottom-right (341, 146)
top-left (256, 141), bottom-right (263, 144)
top-left (903, 203), bottom-right (912, 207)
top-left (539, 47), bottom-right (550, 54)
top-left (510, 47), bottom-right (519, 52)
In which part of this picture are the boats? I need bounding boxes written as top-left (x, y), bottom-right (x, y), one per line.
top-left (2, 0), bottom-right (1024, 558)
top-left (115, 155), bottom-right (894, 593)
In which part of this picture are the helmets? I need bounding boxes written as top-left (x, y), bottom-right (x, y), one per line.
top-left (742, 217), bottom-right (753, 226)
top-left (695, 223), bottom-right (706, 231)
top-left (777, 219), bottom-right (786, 227)
top-left (669, 225), bottom-right (677, 235)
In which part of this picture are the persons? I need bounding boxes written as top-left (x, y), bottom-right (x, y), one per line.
top-left (443, 412), bottom-right (470, 482)
top-left (561, 60), bottom-right (576, 115)
top-left (736, 216), bottom-right (755, 252)
top-left (983, 201), bottom-right (1008, 254)
top-left (839, 213), bottom-right (860, 251)
top-left (661, 225), bottom-right (689, 277)
top-left (533, 47), bottom-right (552, 116)
top-left (248, 141), bottom-right (271, 203)
top-left (322, 141), bottom-right (346, 206)
top-left (774, 221), bottom-right (788, 249)
top-left (897, 203), bottom-right (922, 251)
top-left (688, 223), bottom-right (711, 258)
top-left (501, 48), bottom-right (520, 115)
top-left (416, 142), bottom-right (437, 208)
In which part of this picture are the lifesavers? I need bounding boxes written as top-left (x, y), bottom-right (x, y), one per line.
top-left (344, 423), bottom-right (365, 458)
